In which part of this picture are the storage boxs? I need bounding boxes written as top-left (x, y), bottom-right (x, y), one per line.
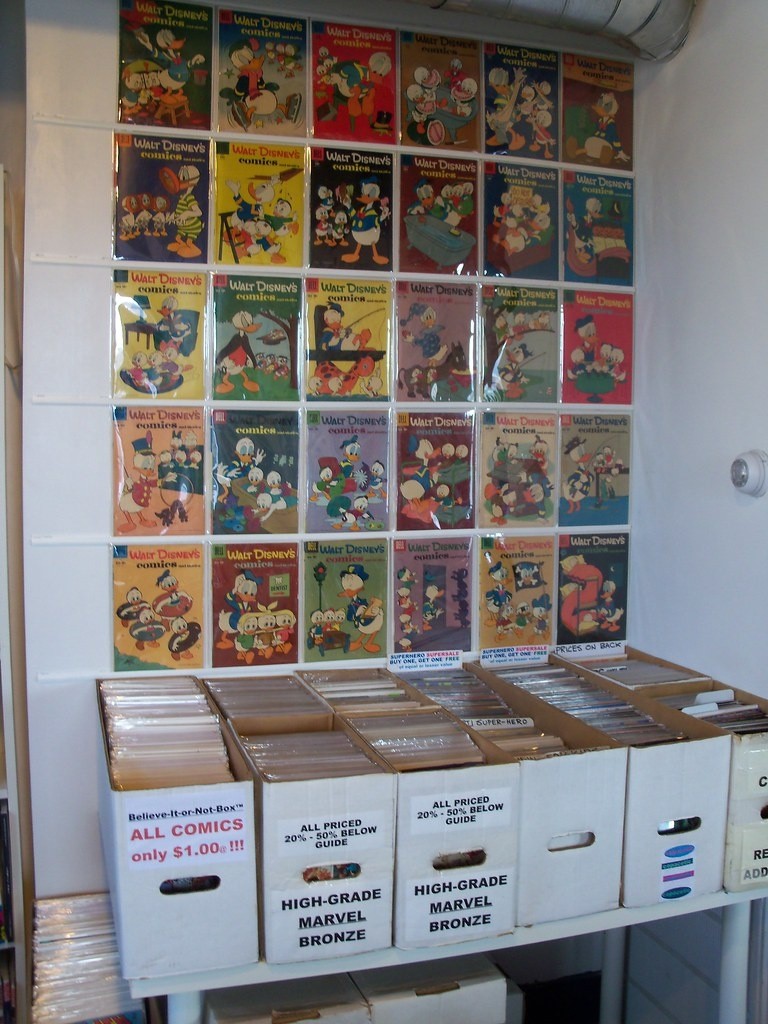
top-left (204, 953), bottom-right (525, 1024)
top-left (96, 645), bottom-right (768, 978)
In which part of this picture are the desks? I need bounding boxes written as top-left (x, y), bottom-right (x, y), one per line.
top-left (130, 888), bottom-right (768, 1024)
